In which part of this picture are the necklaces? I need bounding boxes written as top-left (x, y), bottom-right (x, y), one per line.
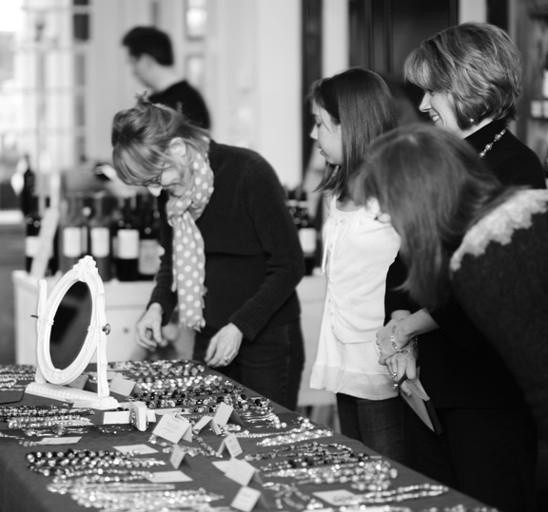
top-left (480, 129), bottom-right (507, 159)
top-left (0, 357), bottom-right (505, 512)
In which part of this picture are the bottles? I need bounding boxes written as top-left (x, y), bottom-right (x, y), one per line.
top-left (23, 190), bottom-right (323, 282)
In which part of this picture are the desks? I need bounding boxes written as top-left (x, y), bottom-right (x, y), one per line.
top-left (11, 270), bottom-right (337, 408)
top-left (0, 358), bottom-right (502, 512)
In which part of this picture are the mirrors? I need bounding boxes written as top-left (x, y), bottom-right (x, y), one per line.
top-left (25, 255), bottom-right (120, 411)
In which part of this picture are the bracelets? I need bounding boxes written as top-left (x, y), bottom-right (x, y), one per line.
top-left (390, 322), bottom-right (421, 353)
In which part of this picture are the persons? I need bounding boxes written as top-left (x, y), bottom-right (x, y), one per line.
top-left (349, 121), bottom-right (547, 511)
top-left (309, 67), bottom-right (403, 468)
top-left (120, 23), bottom-right (209, 134)
top-left (110, 91), bottom-right (307, 411)
top-left (374, 21), bottom-right (547, 511)
